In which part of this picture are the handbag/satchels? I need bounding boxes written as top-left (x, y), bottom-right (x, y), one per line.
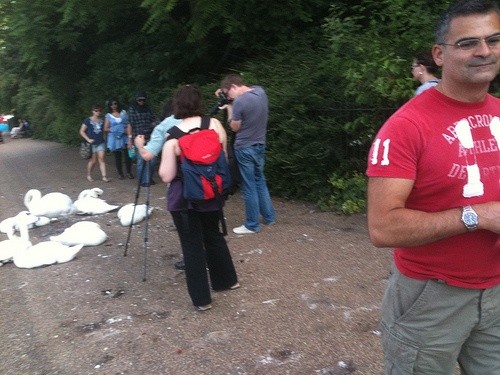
top-left (80, 141), bottom-right (92, 159)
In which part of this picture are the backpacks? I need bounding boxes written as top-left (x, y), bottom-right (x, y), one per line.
top-left (166, 116), bottom-right (239, 203)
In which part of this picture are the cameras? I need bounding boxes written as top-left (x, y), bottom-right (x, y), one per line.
top-left (210, 92), bottom-right (229, 115)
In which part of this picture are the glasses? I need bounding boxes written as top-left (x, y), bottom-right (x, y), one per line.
top-left (436, 35), bottom-right (500, 51)
top-left (225, 88), bottom-right (230, 98)
top-left (409, 65), bottom-right (420, 71)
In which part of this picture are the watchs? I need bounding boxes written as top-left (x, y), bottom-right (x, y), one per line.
top-left (459, 203), bottom-right (479, 232)
top-left (128, 135), bottom-right (132, 138)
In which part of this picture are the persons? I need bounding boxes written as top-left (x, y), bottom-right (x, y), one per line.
top-left (134, 84), bottom-right (185, 270)
top-left (365, 0), bottom-right (500, 375)
top-left (103, 97), bottom-right (135, 179)
top-left (80, 102), bottom-right (113, 182)
top-left (158, 84), bottom-right (240, 311)
top-left (0, 113), bottom-right (29, 143)
top-left (410, 47), bottom-right (442, 96)
top-left (126, 91), bottom-right (160, 187)
top-left (215, 73), bottom-right (275, 235)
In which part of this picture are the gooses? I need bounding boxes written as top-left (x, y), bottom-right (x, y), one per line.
top-left (0, 188), bottom-right (154, 270)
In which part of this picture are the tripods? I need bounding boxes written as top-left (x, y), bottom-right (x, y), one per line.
top-left (122, 126), bottom-right (155, 282)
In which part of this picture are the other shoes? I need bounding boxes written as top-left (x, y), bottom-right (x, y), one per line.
top-left (86, 175), bottom-right (94, 181)
top-left (196, 303), bottom-right (213, 312)
top-left (230, 281), bottom-right (240, 290)
top-left (118, 172), bottom-right (157, 187)
top-left (232, 225), bottom-right (256, 234)
top-left (173, 260), bottom-right (186, 270)
top-left (101, 175), bottom-right (112, 183)
